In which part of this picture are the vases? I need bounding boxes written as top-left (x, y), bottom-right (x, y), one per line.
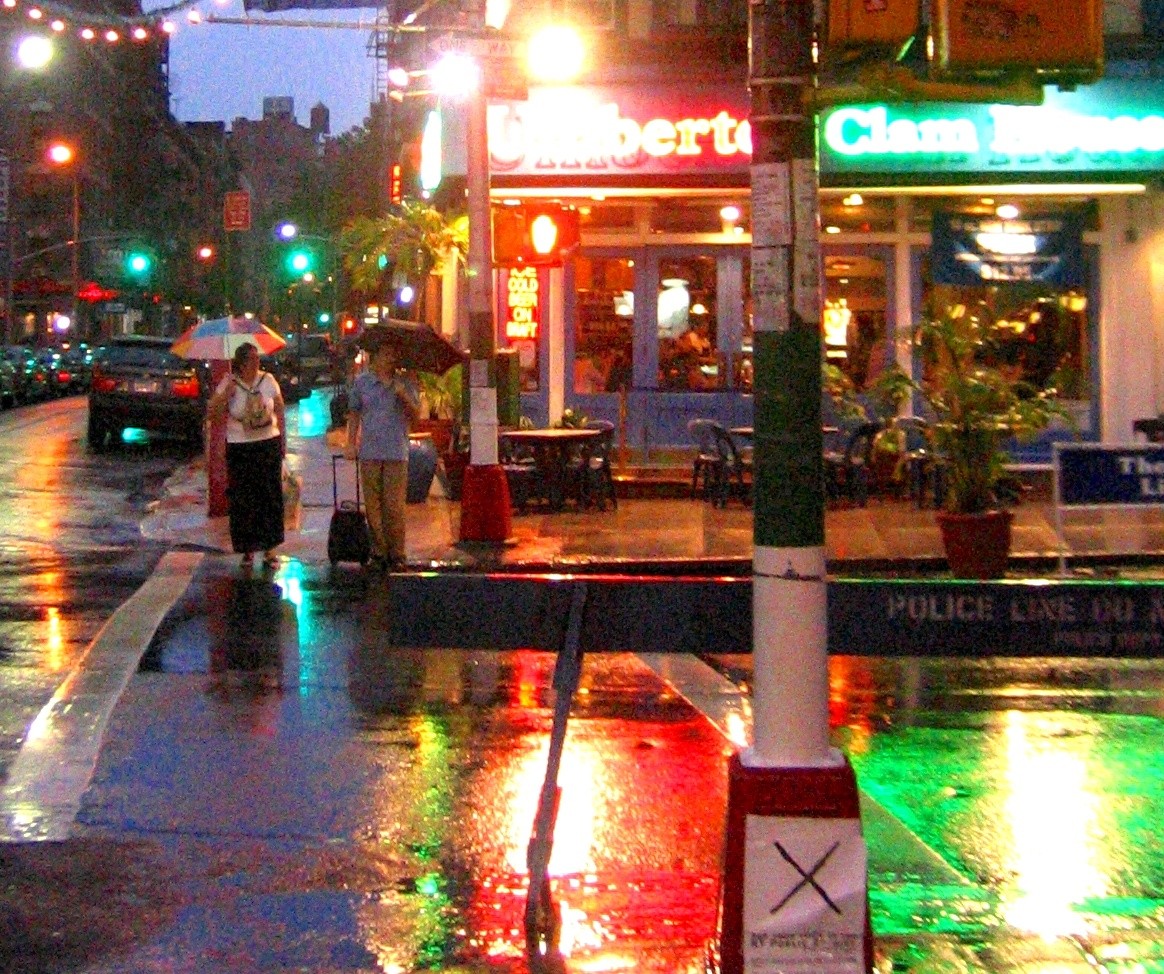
top-left (405, 431), bottom-right (437, 504)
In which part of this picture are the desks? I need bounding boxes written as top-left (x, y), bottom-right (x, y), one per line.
top-left (730, 426), bottom-right (838, 437)
top-left (508, 429), bottom-right (602, 511)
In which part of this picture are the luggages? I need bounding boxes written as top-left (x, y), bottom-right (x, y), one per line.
top-left (328, 454), bottom-right (369, 567)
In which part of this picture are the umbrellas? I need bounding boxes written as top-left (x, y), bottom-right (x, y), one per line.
top-left (355, 318), bottom-right (464, 378)
top-left (169, 316), bottom-right (288, 374)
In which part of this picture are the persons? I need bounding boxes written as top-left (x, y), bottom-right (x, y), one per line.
top-left (846, 295), bottom-right (874, 386)
top-left (343, 342), bottom-right (420, 572)
top-left (206, 341), bottom-right (287, 569)
top-left (659, 315), bottom-right (719, 389)
top-left (574, 340), bottom-right (633, 393)
top-left (1023, 302), bottom-right (1063, 390)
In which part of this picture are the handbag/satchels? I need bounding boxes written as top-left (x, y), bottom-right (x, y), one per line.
top-left (280, 464), bottom-right (302, 529)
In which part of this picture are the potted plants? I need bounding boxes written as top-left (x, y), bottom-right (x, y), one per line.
top-left (883, 302), bottom-right (1081, 577)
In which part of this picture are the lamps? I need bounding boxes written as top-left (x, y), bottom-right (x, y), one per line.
top-left (386, 89), bottom-right (436, 102)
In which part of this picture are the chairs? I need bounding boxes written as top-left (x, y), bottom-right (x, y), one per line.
top-left (823, 421), bottom-right (889, 509)
top-left (688, 416), bottom-right (751, 509)
top-left (498, 420), bottom-right (620, 517)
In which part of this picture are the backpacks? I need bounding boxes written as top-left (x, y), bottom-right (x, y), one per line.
top-left (231, 372), bottom-right (272, 430)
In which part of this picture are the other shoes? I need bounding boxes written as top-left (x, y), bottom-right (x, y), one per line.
top-left (263, 553), bottom-right (279, 569)
top-left (391, 558), bottom-right (407, 572)
top-left (240, 553), bottom-right (254, 568)
